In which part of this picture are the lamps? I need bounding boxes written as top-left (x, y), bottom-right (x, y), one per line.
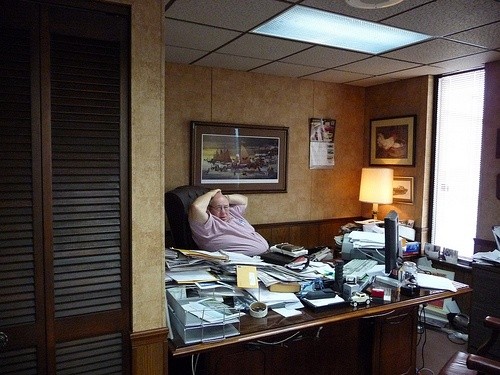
top-left (358, 168), bottom-right (394, 220)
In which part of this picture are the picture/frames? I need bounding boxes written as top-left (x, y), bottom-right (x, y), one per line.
top-left (369, 115), bottom-right (417, 168)
top-left (394, 176), bottom-right (414, 205)
top-left (190, 121), bottom-right (290, 194)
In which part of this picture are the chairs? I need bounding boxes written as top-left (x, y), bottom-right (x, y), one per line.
top-left (165, 185), bottom-right (209, 249)
top-left (439, 316), bottom-right (500, 375)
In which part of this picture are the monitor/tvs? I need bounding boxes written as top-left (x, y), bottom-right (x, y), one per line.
top-left (383, 210), bottom-right (404, 278)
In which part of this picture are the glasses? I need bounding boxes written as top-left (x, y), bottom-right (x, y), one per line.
top-left (209, 204), bottom-right (229, 211)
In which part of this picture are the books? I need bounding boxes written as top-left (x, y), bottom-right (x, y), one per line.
top-left (276, 244), bottom-right (304, 252)
top-left (270, 282), bottom-right (301, 294)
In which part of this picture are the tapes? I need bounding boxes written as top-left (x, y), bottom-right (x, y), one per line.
top-left (248, 301), bottom-right (268, 318)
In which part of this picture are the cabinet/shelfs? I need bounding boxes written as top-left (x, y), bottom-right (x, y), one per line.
top-left (466, 260), bottom-right (500, 362)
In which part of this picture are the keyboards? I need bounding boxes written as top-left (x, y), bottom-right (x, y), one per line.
top-left (343, 258), bottom-right (379, 280)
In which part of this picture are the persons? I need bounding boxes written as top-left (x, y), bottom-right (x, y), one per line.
top-left (190, 189), bottom-right (269, 257)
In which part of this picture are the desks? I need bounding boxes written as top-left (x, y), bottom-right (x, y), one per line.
top-left (169, 256), bottom-right (473, 375)
